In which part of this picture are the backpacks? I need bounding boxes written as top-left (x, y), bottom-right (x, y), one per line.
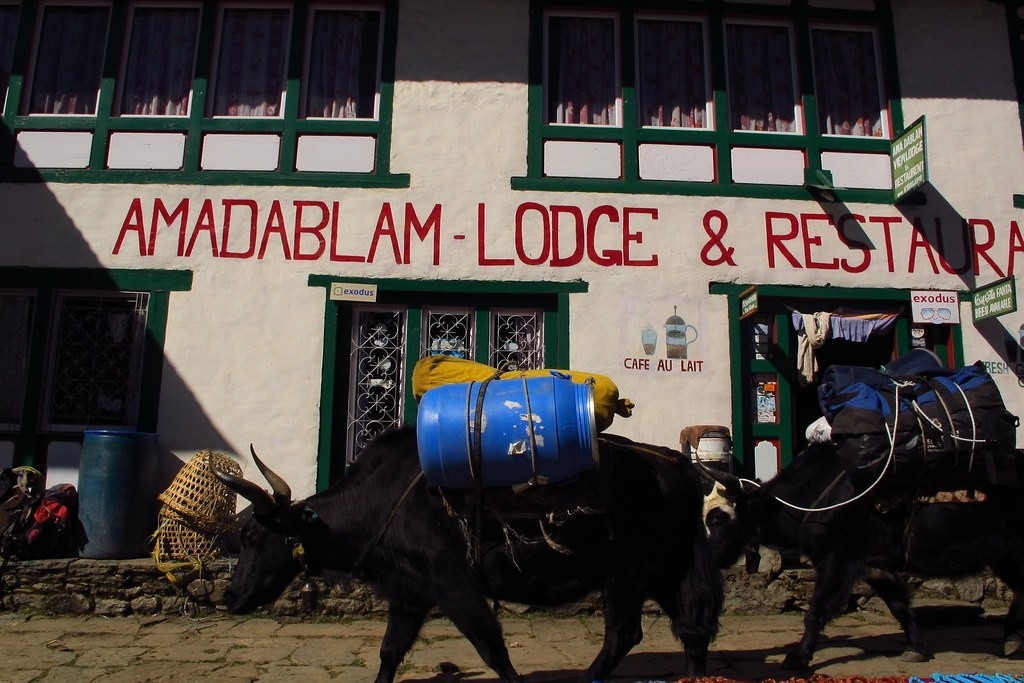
top-left (0, 466), bottom-right (88, 560)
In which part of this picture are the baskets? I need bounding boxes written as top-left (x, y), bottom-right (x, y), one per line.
top-left (151, 449), bottom-right (243, 564)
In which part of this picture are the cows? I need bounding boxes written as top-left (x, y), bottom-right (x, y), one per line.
top-left (206, 424), bottom-right (1024, 683)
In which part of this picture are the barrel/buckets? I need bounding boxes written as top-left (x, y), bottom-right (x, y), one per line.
top-left (80, 428), bottom-right (165, 558)
top-left (679, 424), bottom-right (731, 479)
top-left (414, 375), bottom-right (601, 488)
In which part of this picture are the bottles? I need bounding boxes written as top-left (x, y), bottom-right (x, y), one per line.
top-left (756, 381), bottom-right (768, 422)
